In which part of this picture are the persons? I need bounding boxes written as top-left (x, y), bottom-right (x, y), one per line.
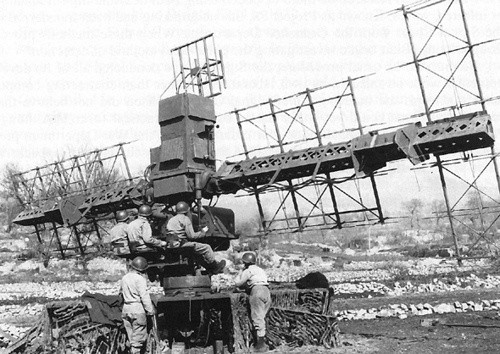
top-left (120, 256), bottom-right (156, 354)
top-left (235, 252), bottom-right (271, 353)
top-left (127, 205), bottom-right (168, 252)
top-left (166, 201), bottom-right (226, 274)
top-left (110, 211), bottom-right (132, 255)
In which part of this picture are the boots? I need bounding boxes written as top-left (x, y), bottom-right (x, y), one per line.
top-left (210, 260), bottom-right (226, 274)
top-left (250, 337), bottom-right (266, 351)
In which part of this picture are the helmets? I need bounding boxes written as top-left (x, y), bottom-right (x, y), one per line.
top-left (131, 256), bottom-right (149, 271)
top-left (242, 253), bottom-right (256, 264)
top-left (138, 205), bottom-right (153, 216)
top-left (116, 210), bottom-right (129, 222)
top-left (176, 201), bottom-right (190, 214)
